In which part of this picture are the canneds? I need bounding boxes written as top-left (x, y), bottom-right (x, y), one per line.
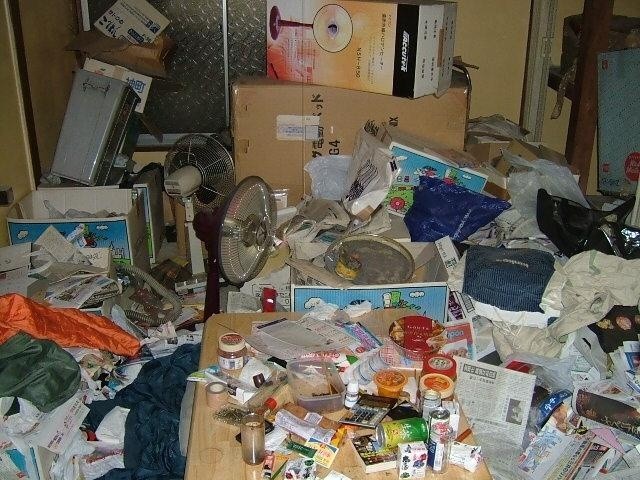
top-left (376, 352), bottom-right (457, 474)
top-left (217, 332), bottom-right (248, 372)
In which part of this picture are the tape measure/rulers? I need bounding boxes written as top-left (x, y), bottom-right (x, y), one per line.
top-left (215, 372), bottom-right (257, 393)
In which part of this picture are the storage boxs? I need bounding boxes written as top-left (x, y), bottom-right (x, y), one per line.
top-left (1, 444), bottom-right (57, 480)
top-left (264, 1), bottom-right (455, 100)
top-left (290, 238), bottom-right (459, 324)
top-left (0, 1), bottom-right (171, 274)
top-left (232, 79), bottom-right (468, 201)
top-left (472, 126), bottom-right (572, 205)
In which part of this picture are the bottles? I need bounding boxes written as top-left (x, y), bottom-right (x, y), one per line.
top-left (344, 379), bottom-right (359, 410)
top-left (420, 389), bottom-right (442, 420)
top-left (428, 422), bottom-right (453, 474)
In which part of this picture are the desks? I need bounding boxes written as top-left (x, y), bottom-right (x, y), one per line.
top-left (182, 308), bottom-right (492, 480)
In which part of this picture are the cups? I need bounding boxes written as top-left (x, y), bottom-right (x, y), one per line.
top-left (240, 413), bottom-right (266, 466)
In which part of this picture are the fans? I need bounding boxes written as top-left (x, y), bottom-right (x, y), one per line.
top-left (160, 134), bottom-right (236, 294)
top-left (190, 176), bottom-right (275, 323)
top-left (269, 6), bottom-right (355, 54)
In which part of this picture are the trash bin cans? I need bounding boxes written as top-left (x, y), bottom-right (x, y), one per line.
top-left (50, 67), bottom-right (140, 188)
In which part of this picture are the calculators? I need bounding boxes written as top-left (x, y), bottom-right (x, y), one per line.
top-left (339, 394), bottom-right (396, 428)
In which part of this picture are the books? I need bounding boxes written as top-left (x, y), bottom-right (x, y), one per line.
top-left (349, 434), bottom-right (400, 475)
top-left (255, 317), bottom-right (325, 347)
